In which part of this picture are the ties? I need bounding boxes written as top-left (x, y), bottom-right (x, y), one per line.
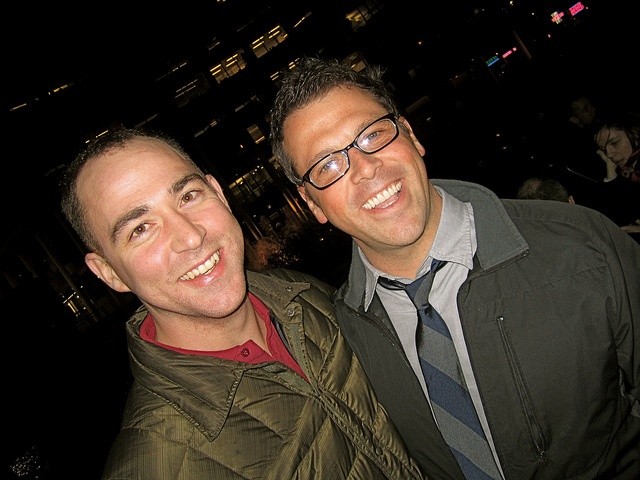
top-left (377, 258), bottom-right (503, 480)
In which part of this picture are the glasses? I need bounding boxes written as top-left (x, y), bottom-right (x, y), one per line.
top-left (301, 112), bottom-right (399, 190)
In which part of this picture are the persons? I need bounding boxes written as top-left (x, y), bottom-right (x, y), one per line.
top-left (269, 62), bottom-right (640, 480)
top-left (59, 126), bottom-right (426, 479)
top-left (517, 177), bottom-right (576, 205)
top-left (591, 112), bottom-right (639, 245)
